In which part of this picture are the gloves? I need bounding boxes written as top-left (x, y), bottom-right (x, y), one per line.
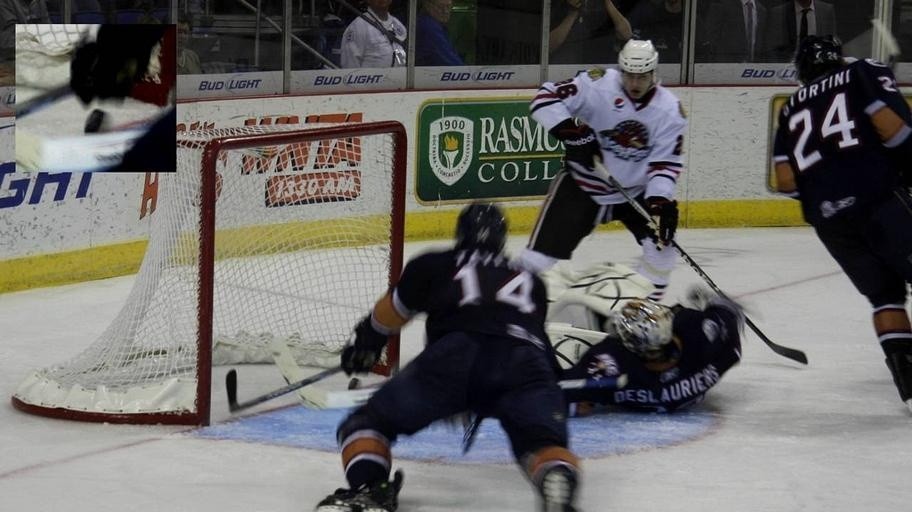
top-left (342, 314), bottom-right (387, 377)
top-left (551, 117), bottom-right (604, 172)
top-left (645, 196), bottom-right (679, 245)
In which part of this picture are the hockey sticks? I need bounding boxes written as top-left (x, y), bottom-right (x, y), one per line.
top-left (224, 362), bottom-right (346, 414)
top-left (595, 157), bottom-right (810, 367)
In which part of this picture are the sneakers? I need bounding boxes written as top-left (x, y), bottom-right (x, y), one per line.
top-left (318, 471), bottom-right (404, 512)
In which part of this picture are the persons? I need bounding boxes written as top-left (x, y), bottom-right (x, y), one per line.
top-left (315, 199), bottom-right (579, 512)
top-left (707, 0), bottom-right (767, 60)
top-left (516, 38), bottom-right (688, 302)
top-left (766, 0), bottom-right (835, 60)
top-left (770, 35), bottom-right (912, 412)
top-left (535, 0), bottom-right (636, 63)
top-left (340, 0), bottom-right (409, 68)
top-left (176, 20), bottom-right (204, 76)
top-left (415, 0), bottom-right (465, 67)
top-left (529, 260), bottom-right (744, 414)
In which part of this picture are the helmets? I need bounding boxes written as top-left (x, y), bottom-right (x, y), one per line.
top-left (613, 301), bottom-right (674, 357)
top-left (794, 35), bottom-right (839, 87)
top-left (456, 202), bottom-right (508, 255)
top-left (823, 34), bottom-right (844, 49)
top-left (617, 39), bottom-right (658, 84)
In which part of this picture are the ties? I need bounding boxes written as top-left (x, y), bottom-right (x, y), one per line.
top-left (799, 8), bottom-right (809, 40)
top-left (746, 1), bottom-right (754, 62)
